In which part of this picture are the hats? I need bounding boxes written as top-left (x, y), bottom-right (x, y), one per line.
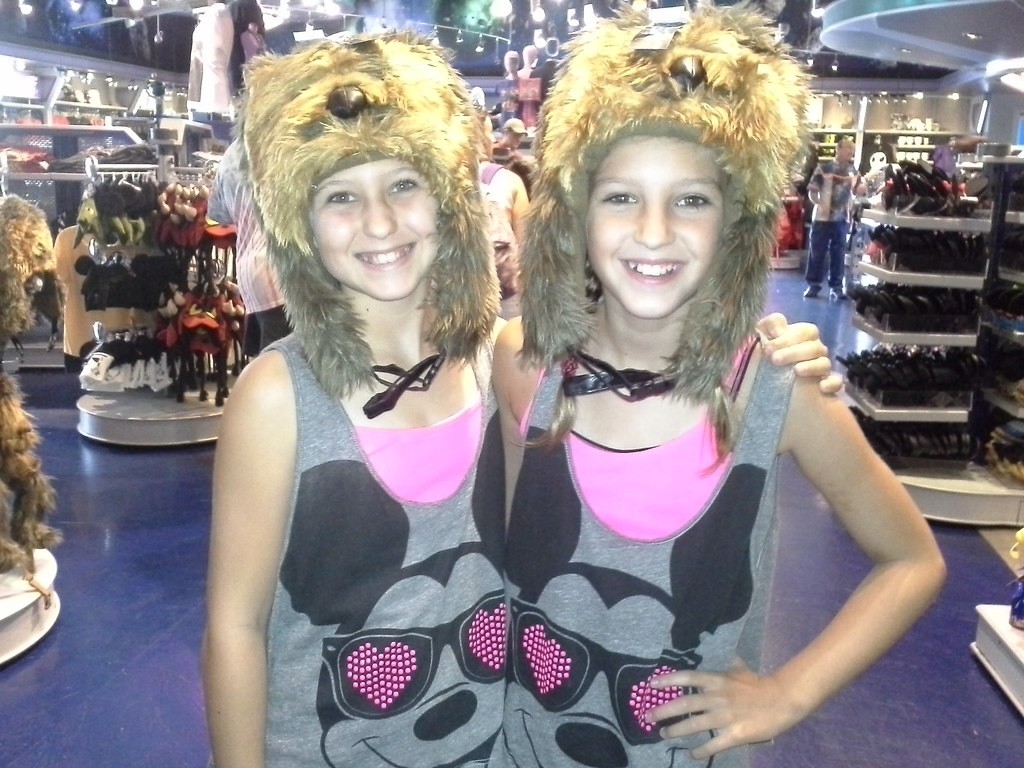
top-left (231, 29), bottom-right (501, 399)
top-left (72, 169), bottom-right (246, 410)
top-left (516, 0), bottom-right (814, 407)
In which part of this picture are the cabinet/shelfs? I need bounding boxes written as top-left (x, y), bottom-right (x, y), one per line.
top-left (772, 132), bottom-right (1024, 483)
top-left (0, 62), bottom-right (245, 408)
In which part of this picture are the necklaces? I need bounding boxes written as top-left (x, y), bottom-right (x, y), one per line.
top-left (248, 30), bottom-right (264, 47)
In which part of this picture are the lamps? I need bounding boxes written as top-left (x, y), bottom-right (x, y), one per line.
top-left (475, 34), bottom-right (486, 52)
top-left (456, 28), bottom-right (463, 44)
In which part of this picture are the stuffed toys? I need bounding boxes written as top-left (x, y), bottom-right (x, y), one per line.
top-left (67, 176), bottom-right (209, 311)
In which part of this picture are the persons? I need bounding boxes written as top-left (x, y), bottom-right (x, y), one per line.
top-left (186, 0), bottom-right (266, 121)
top-left (208, 133), bottom-right (297, 359)
top-left (803, 135), bottom-right (858, 300)
top-left (480, 119), bottom-right (541, 319)
top-left (489, 41), bottom-right (562, 137)
top-left (201, 33), bottom-right (843, 768)
top-left (486, 3), bottom-right (946, 768)
top-left (155, 259), bottom-right (245, 407)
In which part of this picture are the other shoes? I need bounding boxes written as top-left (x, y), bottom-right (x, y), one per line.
top-left (803, 285), bottom-right (822, 297)
top-left (829, 287), bottom-right (847, 300)
